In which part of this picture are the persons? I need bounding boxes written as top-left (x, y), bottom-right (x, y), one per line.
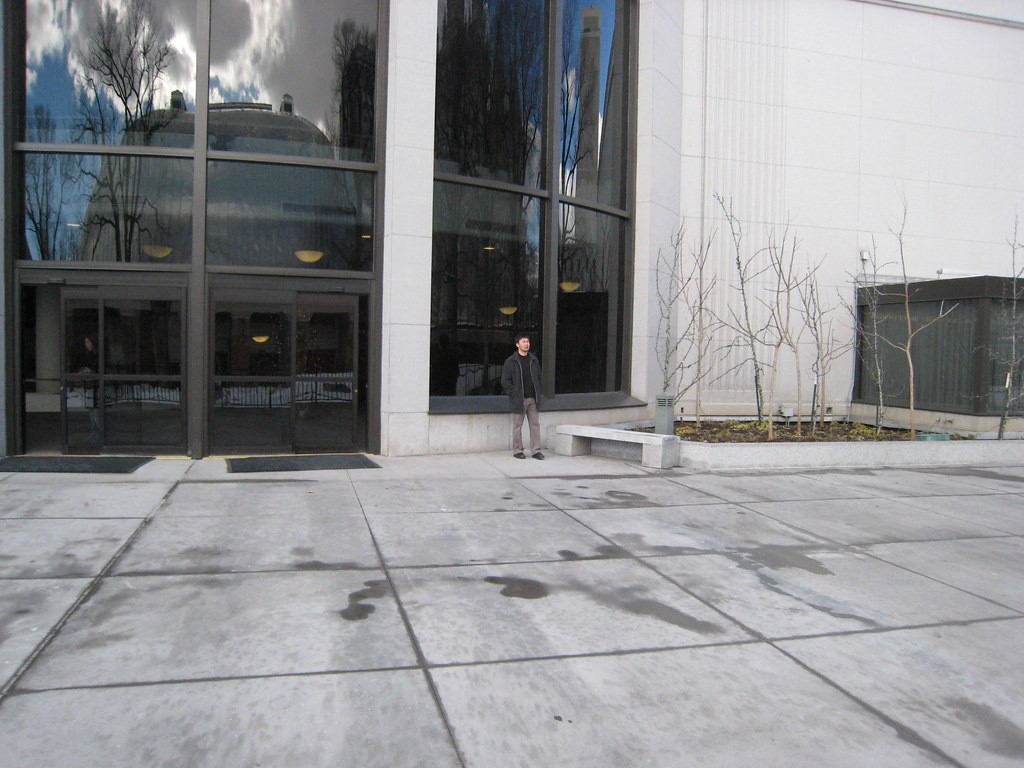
top-left (80, 332), bottom-right (99, 375)
top-left (501, 333), bottom-right (545, 460)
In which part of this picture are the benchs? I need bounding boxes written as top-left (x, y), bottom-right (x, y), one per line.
top-left (554, 425), bottom-right (677, 468)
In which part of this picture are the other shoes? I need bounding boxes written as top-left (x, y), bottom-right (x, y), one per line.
top-left (513, 452), bottom-right (526, 459)
top-left (532, 452), bottom-right (544, 460)
